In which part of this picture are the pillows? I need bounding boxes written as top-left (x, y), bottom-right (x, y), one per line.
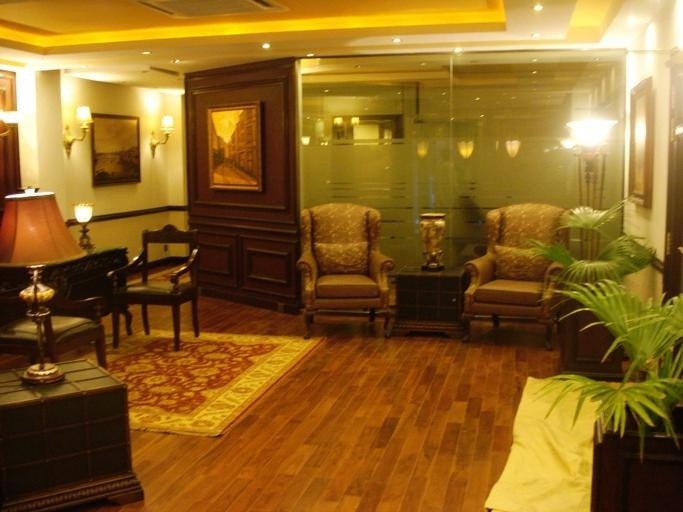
top-left (491, 244), bottom-right (554, 282)
top-left (311, 241), bottom-right (369, 276)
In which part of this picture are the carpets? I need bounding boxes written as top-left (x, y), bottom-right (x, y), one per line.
top-left (65, 323), bottom-right (335, 440)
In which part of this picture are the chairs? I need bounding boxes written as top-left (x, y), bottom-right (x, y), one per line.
top-left (106, 221), bottom-right (204, 351)
top-left (0, 267), bottom-right (113, 369)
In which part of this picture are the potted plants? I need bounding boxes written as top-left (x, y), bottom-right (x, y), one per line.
top-left (536, 275), bottom-right (683, 510)
top-left (527, 194), bottom-right (658, 375)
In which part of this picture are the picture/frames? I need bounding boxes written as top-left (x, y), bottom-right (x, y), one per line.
top-left (204, 99), bottom-right (267, 196)
top-left (89, 113), bottom-right (141, 187)
top-left (626, 74), bottom-right (659, 212)
top-left (0, 69), bottom-right (22, 217)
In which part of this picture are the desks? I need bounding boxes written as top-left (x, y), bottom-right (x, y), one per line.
top-left (0, 245), bottom-right (136, 351)
top-left (395, 266), bottom-right (470, 338)
top-left (0, 358), bottom-right (143, 511)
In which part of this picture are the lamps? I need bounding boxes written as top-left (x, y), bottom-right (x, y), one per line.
top-left (0, 188), bottom-right (89, 388)
top-left (410, 120), bottom-right (524, 163)
top-left (0, 108), bottom-right (23, 140)
top-left (562, 109), bottom-right (621, 265)
top-left (148, 115), bottom-right (177, 159)
top-left (73, 199), bottom-right (98, 252)
top-left (60, 103), bottom-right (95, 159)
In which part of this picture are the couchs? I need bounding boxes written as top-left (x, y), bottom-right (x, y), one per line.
top-left (460, 200), bottom-right (573, 352)
top-left (297, 198), bottom-right (397, 340)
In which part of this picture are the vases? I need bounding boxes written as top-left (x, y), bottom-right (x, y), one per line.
top-left (415, 210), bottom-right (450, 271)
